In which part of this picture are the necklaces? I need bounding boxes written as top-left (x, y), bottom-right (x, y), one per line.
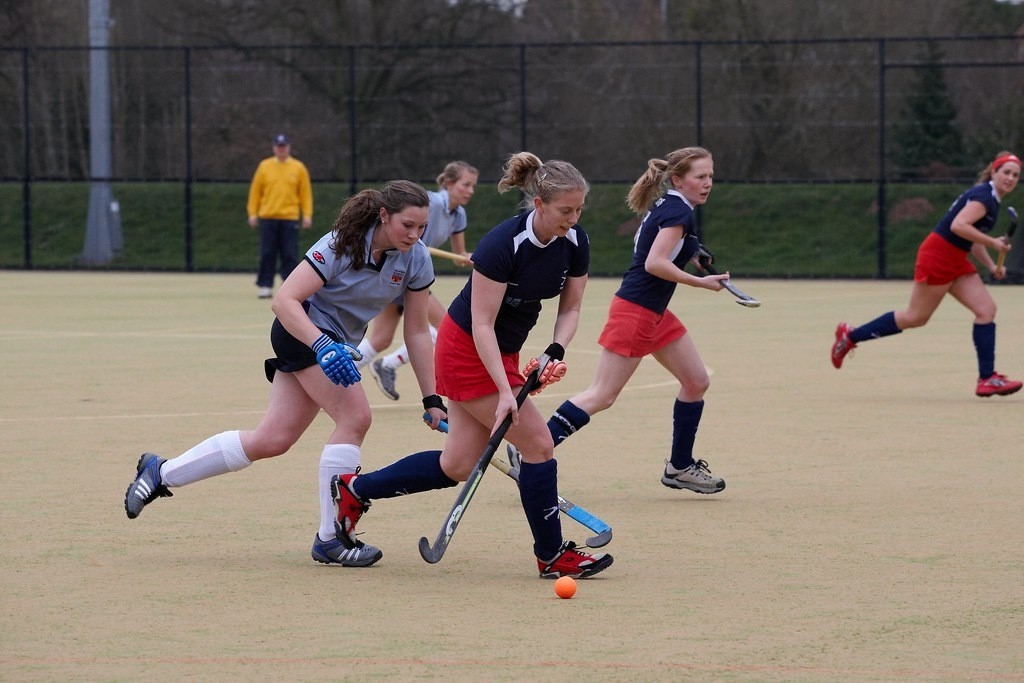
top-left (372, 241), bottom-right (392, 255)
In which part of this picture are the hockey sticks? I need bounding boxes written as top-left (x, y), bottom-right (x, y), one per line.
top-left (995, 206), bottom-right (1019, 275)
top-left (417, 354), bottom-right (614, 565)
top-left (699, 256), bottom-right (762, 309)
top-left (427, 246), bottom-right (468, 265)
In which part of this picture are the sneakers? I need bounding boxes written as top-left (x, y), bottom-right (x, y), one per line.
top-left (506, 443), bottom-right (522, 488)
top-left (537, 539), bottom-right (615, 580)
top-left (310, 532), bottom-right (383, 567)
top-left (975, 372), bottom-right (1022, 398)
top-left (831, 322), bottom-right (858, 369)
top-left (660, 457), bottom-right (726, 494)
top-left (123, 452), bottom-right (174, 519)
top-left (331, 466), bottom-right (372, 550)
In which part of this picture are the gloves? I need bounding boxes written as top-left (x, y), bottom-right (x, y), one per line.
top-left (690, 243), bottom-right (715, 272)
top-left (312, 335), bottom-right (363, 389)
top-left (523, 342), bottom-right (567, 396)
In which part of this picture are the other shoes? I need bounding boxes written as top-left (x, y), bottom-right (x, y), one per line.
top-left (369, 357), bottom-right (400, 400)
top-left (258, 287), bottom-right (273, 298)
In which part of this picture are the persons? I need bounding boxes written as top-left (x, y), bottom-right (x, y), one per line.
top-left (329, 152), bottom-right (615, 579)
top-left (831, 151), bottom-right (1023, 397)
top-left (508, 147), bottom-right (730, 495)
top-left (124, 180), bottom-right (448, 568)
top-left (352, 161), bottom-right (474, 401)
top-left (247, 136), bottom-right (313, 299)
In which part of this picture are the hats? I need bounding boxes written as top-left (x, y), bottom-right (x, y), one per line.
top-left (270, 133), bottom-right (291, 147)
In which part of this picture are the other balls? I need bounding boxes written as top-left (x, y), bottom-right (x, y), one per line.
top-left (554, 576), bottom-right (577, 599)
